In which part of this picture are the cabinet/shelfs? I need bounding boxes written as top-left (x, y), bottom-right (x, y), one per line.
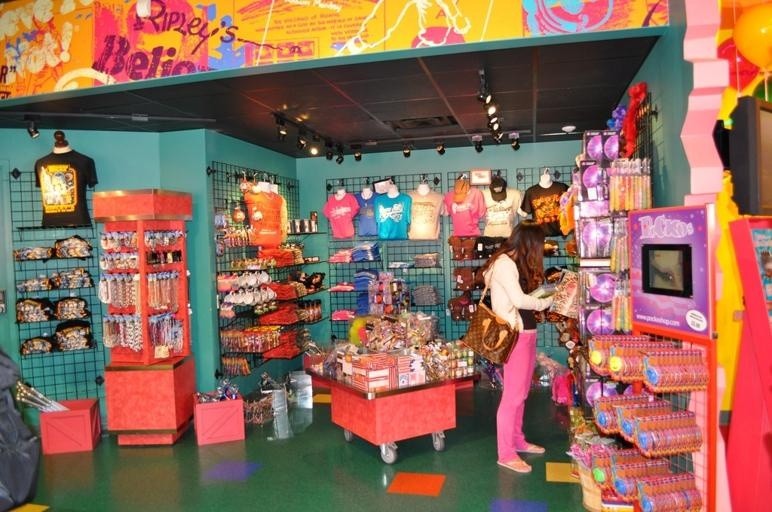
top-left (220, 230), bottom-right (330, 377)
top-left (448, 249), bottom-right (573, 325)
top-left (12, 224), bottom-right (97, 356)
top-left (327, 253), bottom-right (445, 323)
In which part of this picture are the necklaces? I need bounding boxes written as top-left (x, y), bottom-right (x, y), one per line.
top-left (98, 274), bottom-right (184, 354)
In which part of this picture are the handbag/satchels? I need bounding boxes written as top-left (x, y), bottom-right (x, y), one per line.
top-left (461, 301), bottom-right (519, 365)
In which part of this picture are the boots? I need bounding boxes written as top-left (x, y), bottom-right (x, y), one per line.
top-left (453, 237), bottom-right (504, 260)
top-left (448, 296), bottom-right (491, 321)
top-left (454, 266), bottom-right (484, 291)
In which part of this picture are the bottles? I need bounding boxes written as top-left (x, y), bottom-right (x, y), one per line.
top-left (195, 379), bottom-right (239, 403)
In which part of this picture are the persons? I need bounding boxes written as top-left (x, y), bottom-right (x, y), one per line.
top-left (33, 131), bottom-right (98, 228)
top-left (244, 180), bottom-right (289, 247)
top-left (538, 352), bottom-right (573, 406)
top-left (323, 172), bottom-right (571, 243)
top-left (482, 219), bottom-right (558, 474)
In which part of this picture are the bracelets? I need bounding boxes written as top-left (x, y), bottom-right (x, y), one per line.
top-left (97, 231), bottom-right (181, 270)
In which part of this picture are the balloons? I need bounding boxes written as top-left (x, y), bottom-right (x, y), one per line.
top-left (717, 39), bottom-right (760, 91)
top-left (732, 5), bottom-right (772, 72)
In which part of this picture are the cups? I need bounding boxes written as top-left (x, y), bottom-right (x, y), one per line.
top-left (287, 219), bottom-right (318, 233)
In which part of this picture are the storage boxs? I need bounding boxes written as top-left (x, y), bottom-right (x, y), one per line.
top-left (193, 388), bottom-right (249, 447)
top-left (39, 398), bottom-right (103, 457)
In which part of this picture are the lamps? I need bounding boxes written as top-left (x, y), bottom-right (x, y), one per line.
top-left (275, 116), bottom-right (345, 166)
top-left (476, 68), bottom-right (504, 145)
top-left (26, 119), bottom-right (43, 140)
top-left (353, 129), bottom-right (521, 162)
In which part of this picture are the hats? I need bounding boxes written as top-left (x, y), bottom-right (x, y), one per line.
top-left (490, 177), bottom-right (506, 201)
top-left (453, 180), bottom-right (469, 202)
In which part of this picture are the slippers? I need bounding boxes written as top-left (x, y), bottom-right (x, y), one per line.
top-left (516, 443), bottom-right (546, 453)
top-left (497, 458), bottom-right (532, 473)
